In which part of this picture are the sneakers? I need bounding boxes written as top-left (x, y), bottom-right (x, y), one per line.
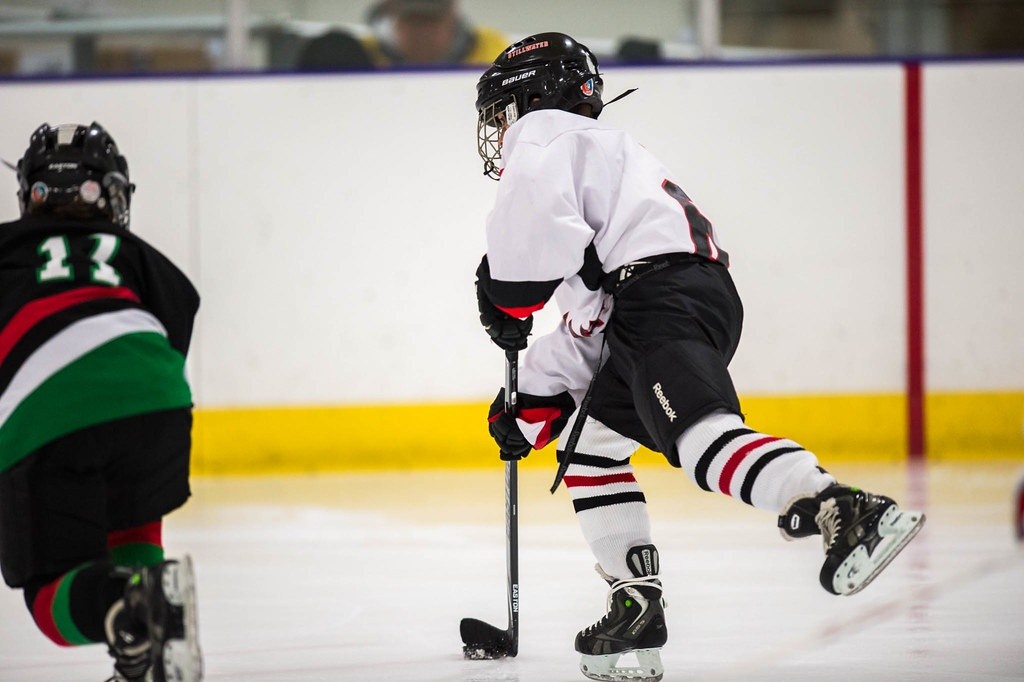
top-left (102, 597), bottom-right (153, 682)
top-left (575, 544), bottom-right (668, 682)
top-left (141, 555), bottom-right (203, 682)
top-left (777, 480), bottom-right (926, 596)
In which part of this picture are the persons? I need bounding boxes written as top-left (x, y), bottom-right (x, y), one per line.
top-left (296, 1), bottom-right (511, 72)
top-left (474, 32), bottom-right (926, 682)
top-left (0, 122), bottom-right (211, 682)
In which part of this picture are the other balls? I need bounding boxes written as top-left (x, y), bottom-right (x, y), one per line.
top-left (462, 641), bottom-right (510, 661)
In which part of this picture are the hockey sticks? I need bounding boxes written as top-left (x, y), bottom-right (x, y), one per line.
top-left (459, 348), bottom-right (523, 658)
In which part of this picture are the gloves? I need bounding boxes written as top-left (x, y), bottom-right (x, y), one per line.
top-left (475, 264), bottom-right (533, 351)
top-left (488, 387), bottom-right (577, 461)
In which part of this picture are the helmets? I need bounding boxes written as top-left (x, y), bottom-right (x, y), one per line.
top-left (476, 32), bottom-right (605, 181)
top-left (16, 121), bottom-right (136, 231)
